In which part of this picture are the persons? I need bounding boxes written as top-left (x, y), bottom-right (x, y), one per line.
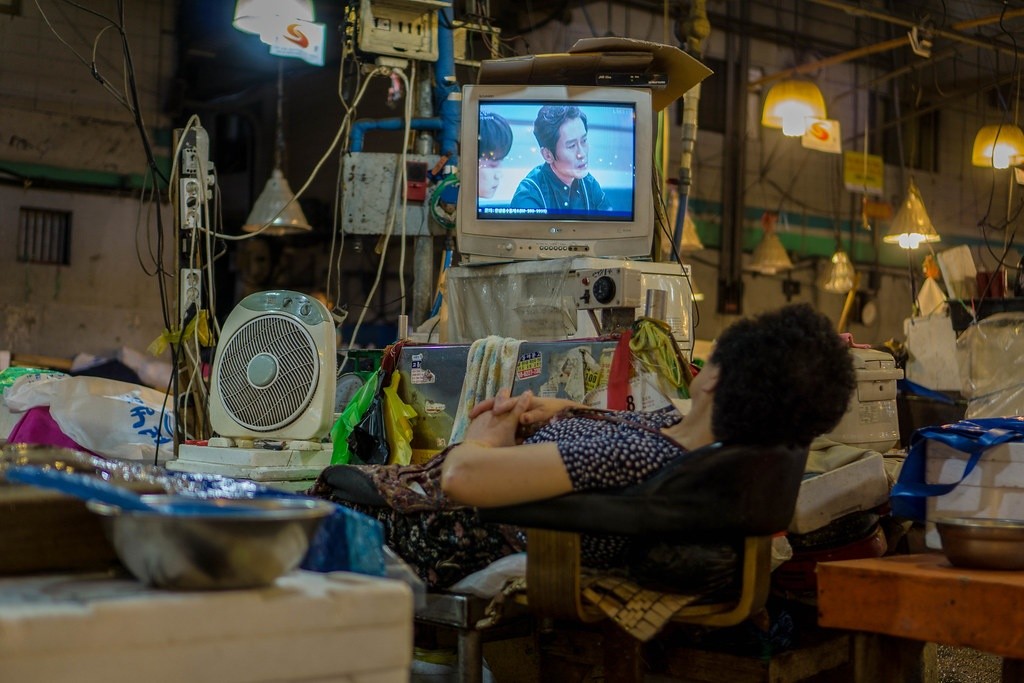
top-left (510, 106), bottom-right (612, 210)
top-left (478, 112), bottom-right (513, 199)
top-left (303, 304), bottom-right (857, 596)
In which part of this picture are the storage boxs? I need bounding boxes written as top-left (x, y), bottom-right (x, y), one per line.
top-left (944, 296), bottom-right (1024, 331)
top-left (924, 437), bottom-right (1024, 551)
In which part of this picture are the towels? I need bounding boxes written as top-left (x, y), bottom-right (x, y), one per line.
top-left (935, 243), bottom-right (978, 301)
top-left (914, 277), bottom-right (948, 318)
top-left (447, 335), bottom-right (528, 447)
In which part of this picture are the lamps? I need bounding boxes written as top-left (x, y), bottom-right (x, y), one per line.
top-left (231, 0), bottom-right (318, 235)
top-left (883, 178), bottom-right (941, 251)
top-left (747, 211), bottom-right (797, 277)
top-left (971, 35), bottom-right (1024, 168)
top-left (659, 178), bottom-right (705, 259)
top-left (758, 0), bottom-right (829, 139)
top-left (815, 234), bottom-right (860, 294)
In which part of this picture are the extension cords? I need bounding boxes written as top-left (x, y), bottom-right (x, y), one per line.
top-left (179, 177), bottom-right (202, 229)
top-left (181, 267), bottom-right (202, 331)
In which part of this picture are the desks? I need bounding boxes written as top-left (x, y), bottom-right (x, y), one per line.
top-left (818, 550), bottom-right (1024, 683)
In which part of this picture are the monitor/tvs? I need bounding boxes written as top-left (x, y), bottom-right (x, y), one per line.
top-left (453, 85), bottom-right (653, 265)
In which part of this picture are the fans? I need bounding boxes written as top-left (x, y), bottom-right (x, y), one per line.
top-left (206, 291), bottom-right (338, 453)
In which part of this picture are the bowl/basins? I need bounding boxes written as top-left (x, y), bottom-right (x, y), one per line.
top-left (928, 517), bottom-right (1024, 571)
top-left (83, 495), bottom-right (332, 590)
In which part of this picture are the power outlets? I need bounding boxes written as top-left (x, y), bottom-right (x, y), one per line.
top-left (180, 268), bottom-right (202, 332)
top-left (180, 177), bottom-right (201, 230)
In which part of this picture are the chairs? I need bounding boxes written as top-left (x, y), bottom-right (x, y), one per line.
top-left (299, 429), bottom-right (811, 683)
